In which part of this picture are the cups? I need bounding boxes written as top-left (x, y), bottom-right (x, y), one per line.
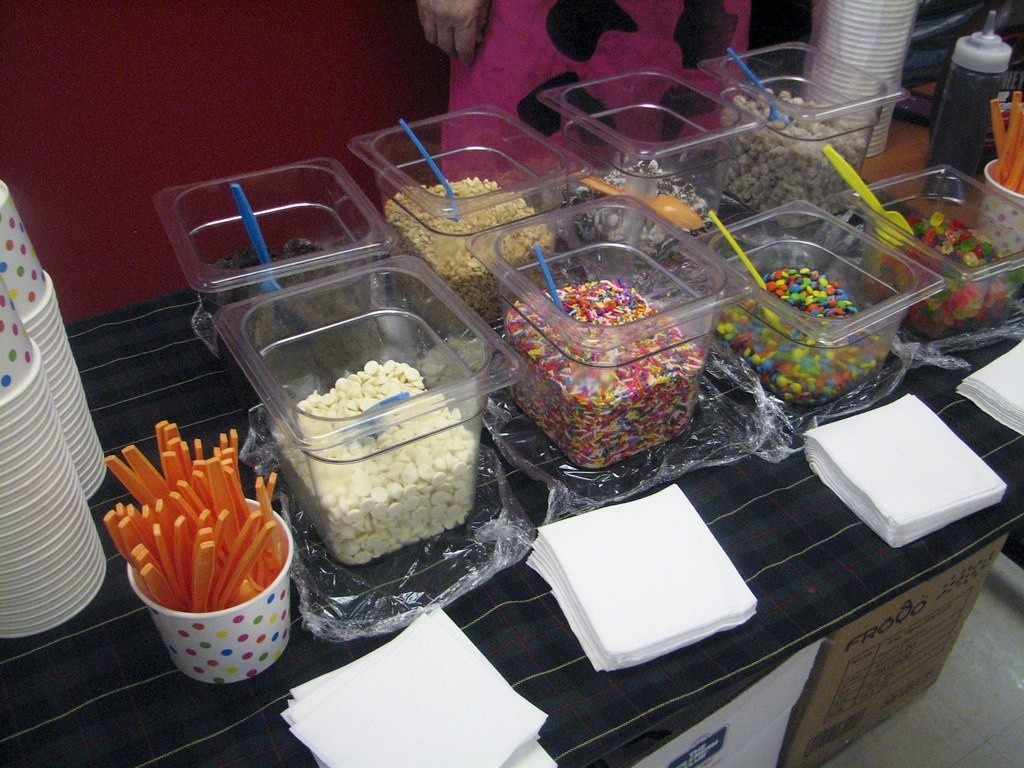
top-left (0, 177), bottom-right (108, 639)
top-left (801, 0), bottom-right (916, 156)
top-left (984, 158), bottom-right (1024, 256)
top-left (124, 496), bottom-right (294, 681)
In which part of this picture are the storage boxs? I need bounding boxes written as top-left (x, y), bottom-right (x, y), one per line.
top-left (826, 161), bottom-right (1024, 344)
top-left (778, 531), bottom-right (1007, 768)
top-left (596, 637), bottom-right (826, 768)
top-left (352, 100), bottom-right (592, 333)
top-left (690, 196), bottom-right (949, 398)
top-left (466, 190), bottom-right (758, 468)
top-left (151, 154), bottom-right (402, 372)
top-left (215, 250), bottom-right (530, 570)
top-left (532, 64), bottom-right (770, 264)
top-left (701, 41), bottom-right (913, 229)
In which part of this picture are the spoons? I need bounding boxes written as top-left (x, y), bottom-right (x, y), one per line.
top-left (580, 173), bottom-right (718, 232)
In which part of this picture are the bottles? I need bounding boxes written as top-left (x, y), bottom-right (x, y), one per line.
top-left (924, 10), bottom-right (1013, 185)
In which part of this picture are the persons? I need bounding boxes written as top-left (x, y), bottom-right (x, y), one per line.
top-left (417, 0), bottom-right (752, 183)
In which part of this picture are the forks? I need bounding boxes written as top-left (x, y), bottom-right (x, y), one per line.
top-left (823, 146), bottom-right (982, 248)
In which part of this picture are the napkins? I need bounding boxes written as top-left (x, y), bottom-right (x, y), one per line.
top-left (282, 340), bottom-right (1024, 768)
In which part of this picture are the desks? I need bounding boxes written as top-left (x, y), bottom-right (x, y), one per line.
top-left (0, 180), bottom-right (1024, 768)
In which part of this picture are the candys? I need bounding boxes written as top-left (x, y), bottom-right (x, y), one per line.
top-left (715, 212), bottom-right (1024, 405)
